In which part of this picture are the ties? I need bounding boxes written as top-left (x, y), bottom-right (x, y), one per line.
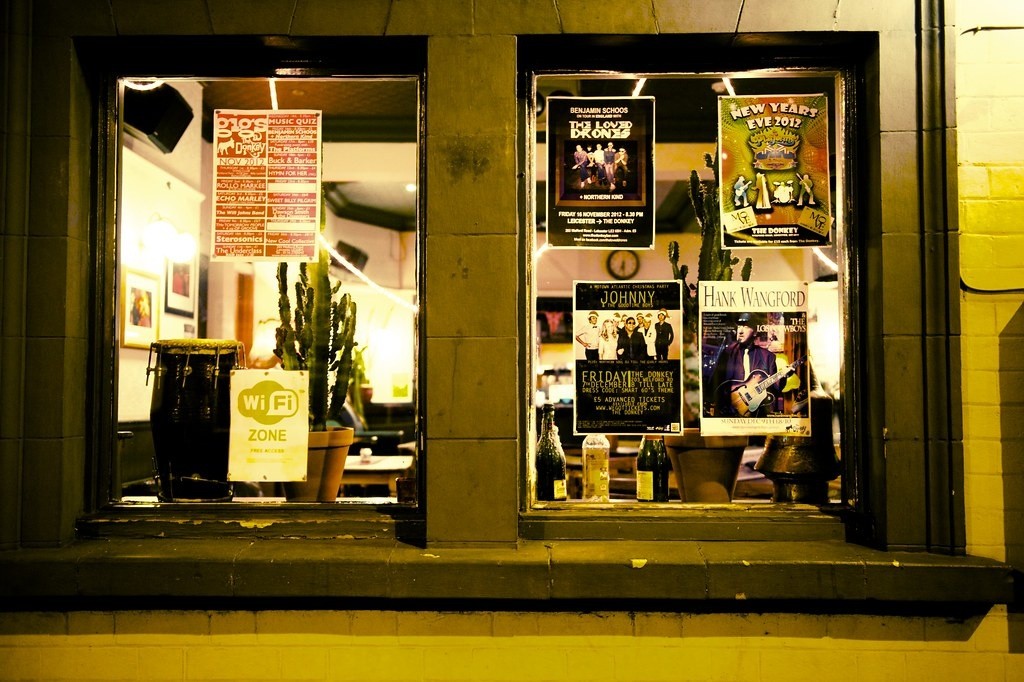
top-left (742, 348), bottom-right (751, 379)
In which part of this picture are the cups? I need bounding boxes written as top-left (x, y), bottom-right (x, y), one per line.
top-left (396, 477), bottom-right (417, 504)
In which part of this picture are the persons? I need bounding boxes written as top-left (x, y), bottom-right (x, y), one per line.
top-left (603, 140), bottom-right (618, 192)
top-left (709, 313), bottom-right (796, 419)
top-left (613, 146), bottom-right (628, 187)
top-left (585, 145), bottom-right (597, 184)
top-left (571, 144), bottom-right (592, 188)
top-left (575, 304), bottom-right (675, 361)
top-left (591, 143), bottom-right (607, 186)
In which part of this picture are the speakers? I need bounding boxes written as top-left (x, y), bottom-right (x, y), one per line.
top-left (120, 80), bottom-right (194, 154)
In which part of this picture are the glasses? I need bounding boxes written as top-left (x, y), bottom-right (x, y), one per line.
top-left (627, 322), bottom-right (636, 325)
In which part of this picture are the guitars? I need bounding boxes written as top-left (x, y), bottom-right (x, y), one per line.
top-left (715, 357), bottom-right (804, 417)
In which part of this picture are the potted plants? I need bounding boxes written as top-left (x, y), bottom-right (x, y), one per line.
top-left (268, 189), bottom-right (358, 501)
top-left (662, 132), bottom-right (753, 504)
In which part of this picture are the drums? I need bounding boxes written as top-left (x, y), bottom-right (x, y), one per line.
top-left (149, 339), bottom-right (234, 502)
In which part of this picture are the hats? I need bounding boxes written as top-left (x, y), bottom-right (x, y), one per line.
top-left (734, 312), bottom-right (759, 329)
top-left (587, 310), bottom-right (599, 319)
top-left (612, 312), bottom-right (652, 321)
top-left (658, 307), bottom-right (672, 319)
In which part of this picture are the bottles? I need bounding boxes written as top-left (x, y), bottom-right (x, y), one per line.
top-left (536, 403), bottom-right (567, 502)
top-left (635, 436), bottom-right (659, 501)
top-left (582, 434), bottom-right (609, 502)
top-left (655, 440), bottom-right (669, 502)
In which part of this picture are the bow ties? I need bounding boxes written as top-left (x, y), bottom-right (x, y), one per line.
top-left (593, 326), bottom-right (597, 328)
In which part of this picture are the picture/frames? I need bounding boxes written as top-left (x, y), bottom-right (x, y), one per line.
top-left (119, 266), bottom-right (162, 351)
top-left (166, 255), bottom-right (197, 319)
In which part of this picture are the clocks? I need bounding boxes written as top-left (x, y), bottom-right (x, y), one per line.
top-left (605, 251), bottom-right (640, 279)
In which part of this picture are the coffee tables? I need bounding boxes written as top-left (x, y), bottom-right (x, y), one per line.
top-left (339, 454), bottom-right (415, 499)
